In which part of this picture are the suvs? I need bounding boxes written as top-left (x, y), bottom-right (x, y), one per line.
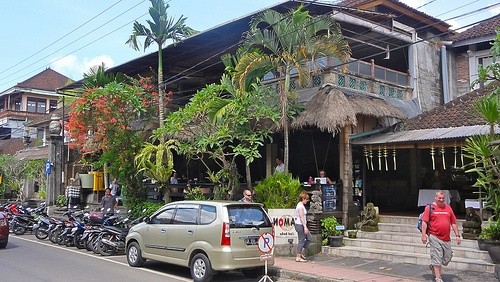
top-left (125, 200), bottom-right (275, 282)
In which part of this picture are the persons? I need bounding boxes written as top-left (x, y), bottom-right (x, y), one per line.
top-left (274, 157), bottom-right (286, 174)
top-left (317, 171), bottom-right (333, 183)
top-left (100, 178), bottom-right (122, 218)
top-left (238, 189), bottom-right (255, 203)
top-left (421, 191), bottom-right (462, 282)
top-left (294, 191), bottom-right (313, 261)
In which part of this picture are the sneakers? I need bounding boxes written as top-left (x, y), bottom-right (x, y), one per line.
top-left (295, 255), bottom-right (307, 263)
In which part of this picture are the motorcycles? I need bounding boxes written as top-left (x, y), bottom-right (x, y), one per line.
top-left (4, 201), bottom-right (146, 255)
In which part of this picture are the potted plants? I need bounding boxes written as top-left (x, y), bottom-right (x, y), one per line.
top-left (484, 219), bottom-right (500, 263)
top-left (320, 215), bottom-right (344, 247)
top-left (476, 228), bottom-right (493, 250)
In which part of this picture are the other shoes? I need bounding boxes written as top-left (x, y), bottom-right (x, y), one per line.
top-left (435, 276), bottom-right (443, 282)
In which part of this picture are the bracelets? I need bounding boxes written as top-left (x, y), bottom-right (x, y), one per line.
top-left (456, 235), bottom-right (460, 237)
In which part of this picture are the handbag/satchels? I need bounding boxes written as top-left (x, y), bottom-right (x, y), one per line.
top-left (417, 203), bottom-right (428, 235)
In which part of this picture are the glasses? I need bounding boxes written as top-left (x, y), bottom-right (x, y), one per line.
top-left (246, 194), bottom-right (251, 197)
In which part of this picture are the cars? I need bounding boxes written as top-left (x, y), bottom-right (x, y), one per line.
top-left (0, 205), bottom-right (9, 250)
